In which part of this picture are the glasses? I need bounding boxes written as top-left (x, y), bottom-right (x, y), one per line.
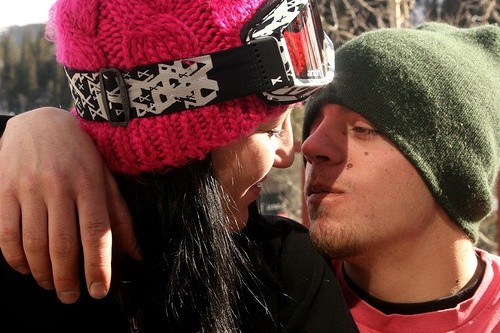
top-left (62, 0), bottom-right (336, 128)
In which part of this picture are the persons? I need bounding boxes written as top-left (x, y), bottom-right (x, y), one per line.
top-left (1, 18), bottom-right (500, 333)
top-left (0, 0), bottom-right (361, 333)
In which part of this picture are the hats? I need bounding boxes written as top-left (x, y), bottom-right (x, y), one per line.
top-left (45, 0), bottom-right (301, 175)
top-left (300, 21), bottom-right (500, 246)
